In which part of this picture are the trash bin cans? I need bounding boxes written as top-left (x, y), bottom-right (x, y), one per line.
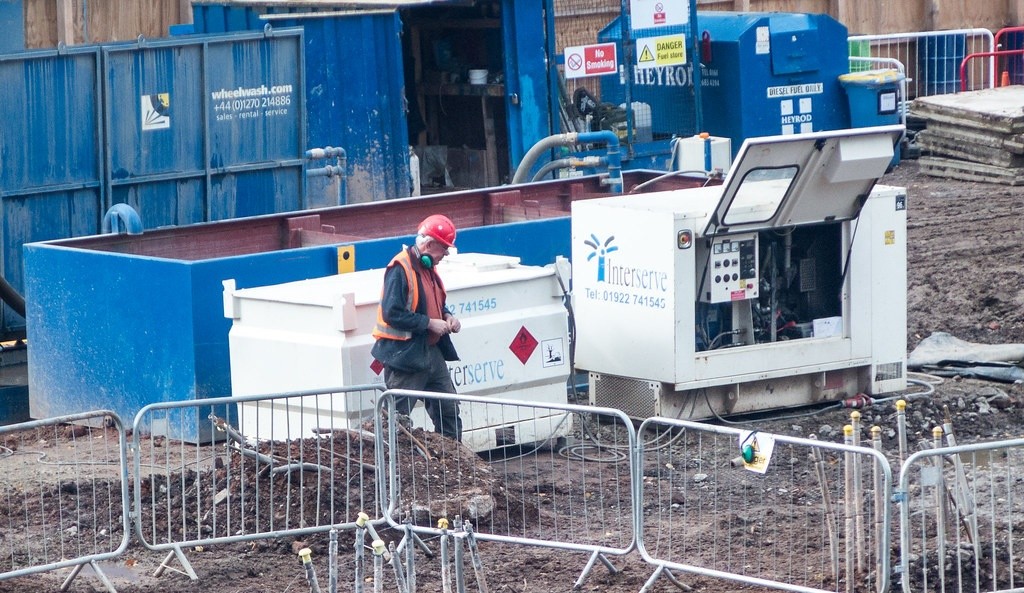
top-left (838, 67), bottom-right (906, 165)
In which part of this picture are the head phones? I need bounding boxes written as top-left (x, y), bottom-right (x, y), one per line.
top-left (414, 244), bottom-right (434, 269)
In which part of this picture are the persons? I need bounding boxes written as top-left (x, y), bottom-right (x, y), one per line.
top-left (372, 214), bottom-right (462, 446)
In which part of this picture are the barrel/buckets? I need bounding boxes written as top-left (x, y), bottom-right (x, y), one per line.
top-left (469, 69), bottom-right (489, 85)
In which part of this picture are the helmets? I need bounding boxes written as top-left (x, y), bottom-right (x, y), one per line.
top-left (417, 215), bottom-right (457, 248)
